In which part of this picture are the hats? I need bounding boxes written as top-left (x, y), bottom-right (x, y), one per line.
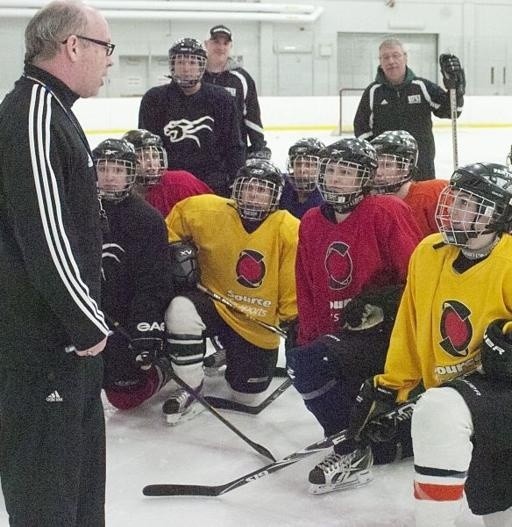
top-left (209, 26), bottom-right (231, 42)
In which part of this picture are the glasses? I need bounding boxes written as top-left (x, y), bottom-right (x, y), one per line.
top-left (63, 34), bottom-right (115, 56)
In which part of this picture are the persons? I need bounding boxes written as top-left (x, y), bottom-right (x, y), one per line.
top-left (285, 137), bottom-right (426, 492)
top-left (348, 160), bottom-right (511, 517)
top-left (0, 0), bottom-right (118, 527)
top-left (89, 24), bottom-right (512, 427)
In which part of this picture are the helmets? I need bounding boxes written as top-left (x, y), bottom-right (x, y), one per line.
top-left (92, 130), bottom-right (163, 191)
top-left (232, 130), bottom-right (419, 211)
top-left (170, 39), bottom-right (207, 76)
top-left (450, 163), bottom-right (512, 232)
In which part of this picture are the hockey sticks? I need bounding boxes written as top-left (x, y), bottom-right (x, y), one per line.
top-left (104, 312), bottom-right (275, 462)
top-left (200, 376), bottom-right (293, 415)
top-left (140, 366), bottom-right (484, 496)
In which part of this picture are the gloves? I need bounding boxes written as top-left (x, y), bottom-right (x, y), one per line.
top-left (168, 242), bottom-right (202, 287)
top-left (347, 379), bottom-right (398, 444)
top-left (481, 319), bottom-right (510, 380)
top-left (127, 293), bottom-right (167, 366)
top-left (440, 53), bottom-right (465, 93)
top-left (341, 290), bottom-right (395, 331)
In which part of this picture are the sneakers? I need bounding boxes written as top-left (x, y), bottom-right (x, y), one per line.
top-left (309, 440), bottom-right (375, 484)
top-left (203, 346), bottom-right (228, 367)
top-left (163, 386), bottom-right (204, 412)
